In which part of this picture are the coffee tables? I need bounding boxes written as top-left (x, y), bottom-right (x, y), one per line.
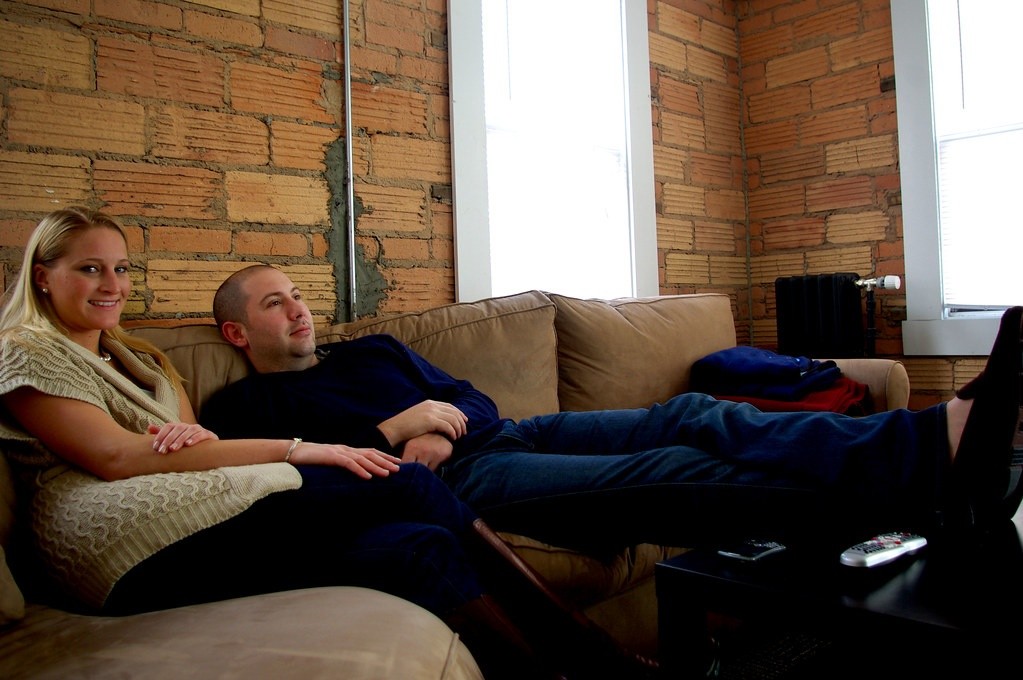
top-left (654, 548), bottom-right (1023, 680)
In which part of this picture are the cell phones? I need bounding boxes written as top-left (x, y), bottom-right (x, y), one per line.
top-left (718, 542), bottom-right (787, 561)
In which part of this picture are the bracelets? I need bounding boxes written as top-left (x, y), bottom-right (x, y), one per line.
top-left (285, 438), bottom-right (302, 462)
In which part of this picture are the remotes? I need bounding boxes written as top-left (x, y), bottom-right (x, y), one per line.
top-left (840, 532), bottom-right (927, 569)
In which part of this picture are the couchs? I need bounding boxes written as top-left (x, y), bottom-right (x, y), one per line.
top-left (0, 289), bottom-right (910, 680)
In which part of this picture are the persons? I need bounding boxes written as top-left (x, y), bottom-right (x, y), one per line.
top-left (196, 265), bottom-right (1023, 546)
top-left (0, 205), bottom-right (728, 680)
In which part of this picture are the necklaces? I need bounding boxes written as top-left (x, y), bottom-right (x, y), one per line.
top-left (100, 350), bottom-right (111, 362)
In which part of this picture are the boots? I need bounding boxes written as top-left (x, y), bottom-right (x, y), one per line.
top-left (443, 593), bottom-right (569, 680)
top-left (459, 518), bottom-right (720, 680)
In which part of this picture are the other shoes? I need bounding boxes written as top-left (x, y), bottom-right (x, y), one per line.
top-left (953, 306), bottom-right (1023, 527)
